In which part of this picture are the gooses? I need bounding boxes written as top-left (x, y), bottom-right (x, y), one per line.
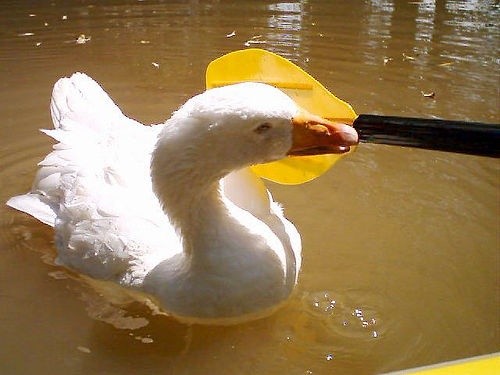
top-left (6, 72), bottom-right (359, 324)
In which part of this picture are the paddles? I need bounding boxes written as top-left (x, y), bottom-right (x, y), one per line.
top-left (205, 48), bottom-right (500, 187)
